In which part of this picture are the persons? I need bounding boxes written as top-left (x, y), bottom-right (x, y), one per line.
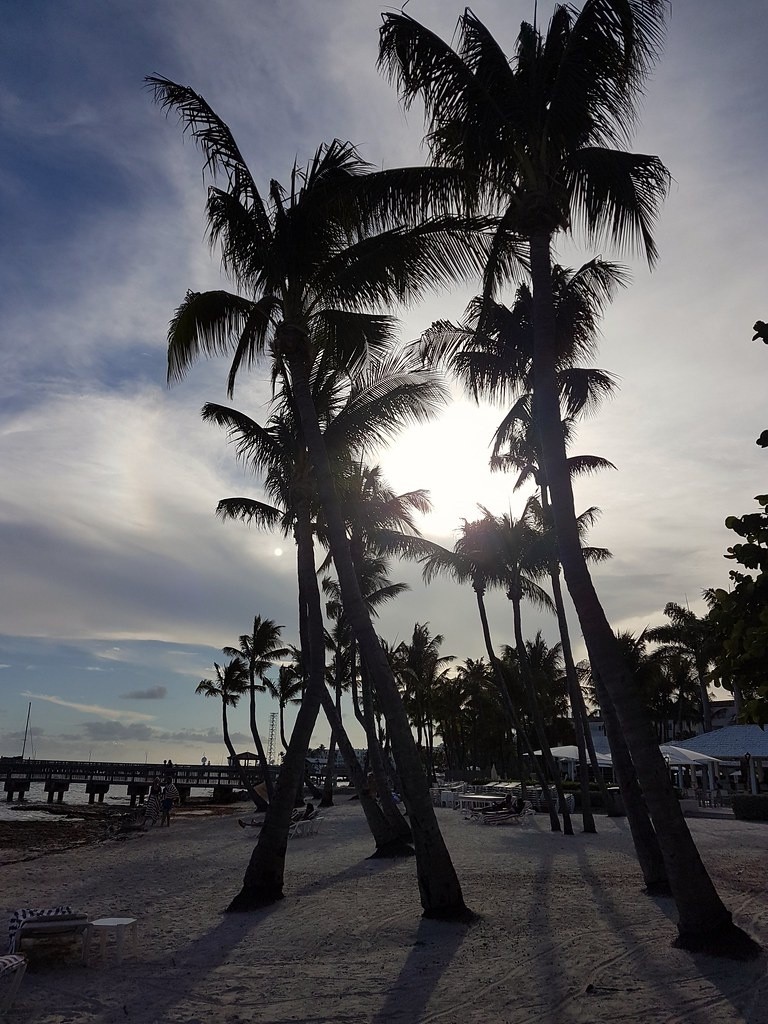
top-left (714, 776), bottom-right (720, 789)
top-left (469, 795), bottom-right (512, 813)
top-left (238, 803), bottom-right (314, 828)
top-left (163, 760), bottom-right (172, 782)
top-left (141, 778), bottom-right (161, 826)
top-left (482, 798), bottom-right (524, 816)
top-left (560, 762), bottom-right (569, 782)
top-left (160, 777), bottom-right (180, 827)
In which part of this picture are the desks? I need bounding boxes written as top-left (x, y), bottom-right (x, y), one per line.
top-left (84, 918), bottom-right (139, 965)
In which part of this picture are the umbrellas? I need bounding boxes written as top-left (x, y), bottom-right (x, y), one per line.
top-left (582, 752), bottom-right (621, 783)
top-left (729, 770), bottom-right (742, 775)
top-left (522, 745), bottom-right (610, 782)
top-left (659, 744), bottom-right (720, 765)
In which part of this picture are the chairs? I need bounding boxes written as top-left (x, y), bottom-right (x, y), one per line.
top-left (429, 780), bottom-right (575, 826)
top-left (254, 808), bottom-right (324, 839)
top-left (695, 789), bottom-right (744, 809)
top-left (0, 905), bottom-right (91, 1016)
